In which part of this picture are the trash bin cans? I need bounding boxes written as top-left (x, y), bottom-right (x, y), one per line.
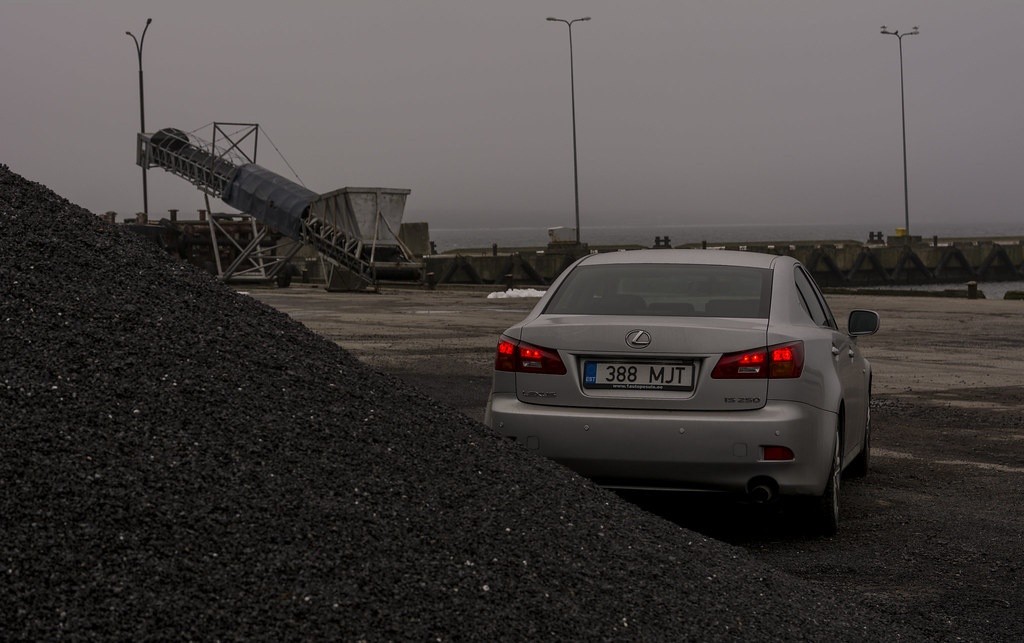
top-left (546, 242), bottom-right (589, 288)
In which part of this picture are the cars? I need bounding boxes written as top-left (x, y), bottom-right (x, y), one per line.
top-left (486, 248), bottom-right (880, 537)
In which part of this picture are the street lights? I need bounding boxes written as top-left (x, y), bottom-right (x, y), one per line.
top-left (125, 18), bottom-right (152, 225)
top-left (880, 25), bottom-right (920, 247)
top-left (546, 16), bottom-right (591, 244)
